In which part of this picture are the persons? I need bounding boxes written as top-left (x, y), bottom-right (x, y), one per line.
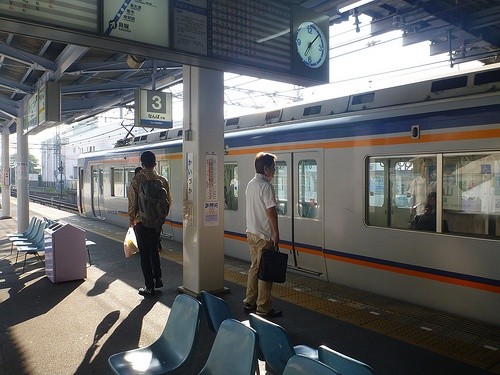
top-left (128, 152), bottom-right (172, 296)
top-left (242, 152), bottom-right (282, 317)
top-left (411, 194), bottom-right (449, 233)
top-left (407, 161), bottom-right (437, 219)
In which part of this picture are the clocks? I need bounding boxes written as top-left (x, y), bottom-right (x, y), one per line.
top-left (295, 21), bottom-right (326, 67)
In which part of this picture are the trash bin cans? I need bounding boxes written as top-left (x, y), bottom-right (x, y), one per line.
top-left (44, 220), bottom-right (87, 283)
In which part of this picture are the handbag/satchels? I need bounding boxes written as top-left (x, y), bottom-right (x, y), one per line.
top-left (257, 244), bottom-right (288, 283)
top-left (124, 226), bottom-right (139, 258)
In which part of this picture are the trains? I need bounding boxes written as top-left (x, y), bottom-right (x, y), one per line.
top-left (77, 67), bottom-right (500, 329)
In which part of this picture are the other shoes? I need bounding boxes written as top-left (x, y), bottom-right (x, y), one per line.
top-left (256, 308), bottom-right (282, 318)
top-left (245, 302), bottom-right (257, 308)
top-left (153, 279), bottom-right (163, 288)
top-left (139, 286), bottom-right (155, 295)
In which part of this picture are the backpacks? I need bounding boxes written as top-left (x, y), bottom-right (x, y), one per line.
top-left (131, 175), bottom-right (170, 227)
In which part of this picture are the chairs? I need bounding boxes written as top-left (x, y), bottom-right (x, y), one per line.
top-left (108, 289), bottom-right (378, 375)
top-left (6, 215), bottom-right (96, 276)
